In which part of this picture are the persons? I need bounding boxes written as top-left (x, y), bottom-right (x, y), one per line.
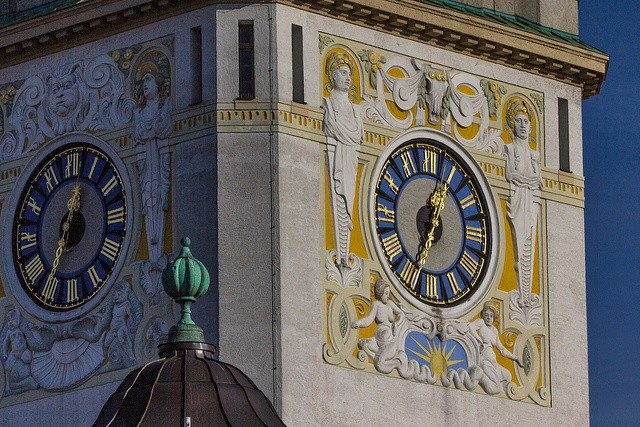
top-left (1, 304), bottom-right (33, 398)
top-left (355, 278), bottom-right (406, 376)
top-left (505, 97), bottom-right (542, 306)
top-left (41, 67), bottom-right (86, 134)
top-left (467, 302), bottom-right (524, 393)
top-left (323, 50), bottom-right (363, 267)
top-left (103, 283), bottom-right (133, 366)
top-left (132, 62), bottom-right (173, 263)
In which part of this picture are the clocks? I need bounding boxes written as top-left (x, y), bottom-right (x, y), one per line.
top-left (1, 133), bottom-right (136, 330)
top-left (358, 128), bottom-right (506, 322)
